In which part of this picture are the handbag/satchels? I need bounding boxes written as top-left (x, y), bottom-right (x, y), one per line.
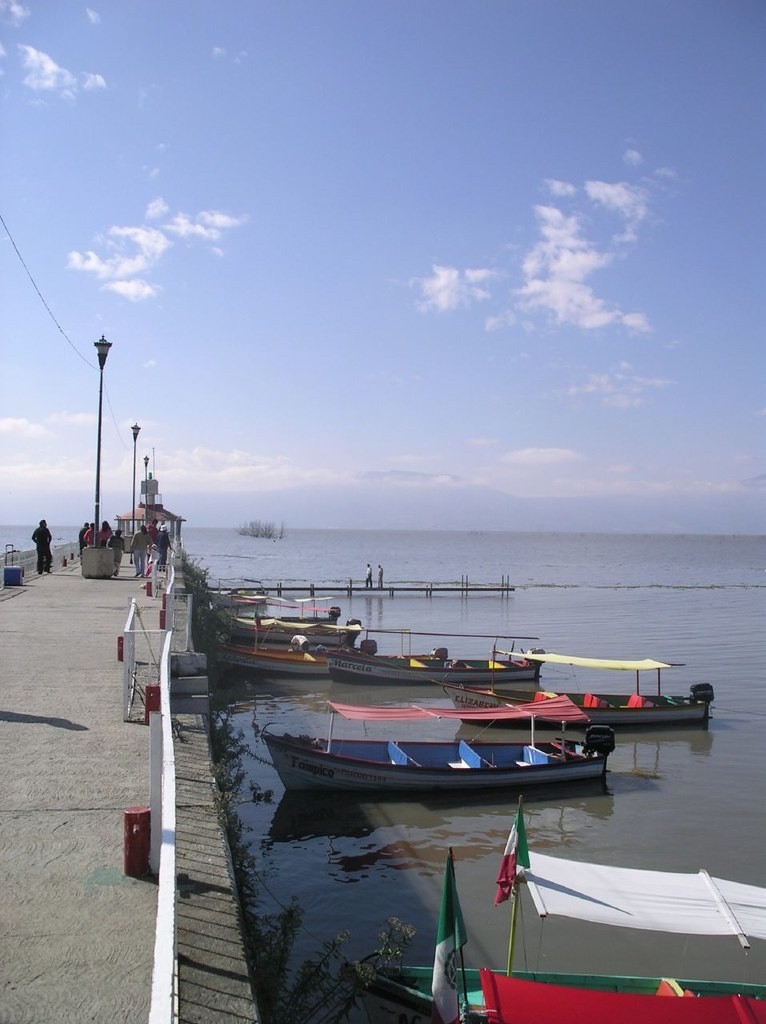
top-left (130, 552), bottom-right (133, 564)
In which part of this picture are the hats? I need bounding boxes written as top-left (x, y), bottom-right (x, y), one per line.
top-left (160, 525), bottom-right (167, 531)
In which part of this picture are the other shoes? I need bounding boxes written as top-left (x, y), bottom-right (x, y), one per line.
top-left (44, 570), bottom-right (52, 573)
top-left (135, 572), bottom-right (141, 577)
top-left (38, 571), bottom-right (42, 574)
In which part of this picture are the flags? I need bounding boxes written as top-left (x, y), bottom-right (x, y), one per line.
top-left (431, 854), bottom-right (468, 1024)
top-left (494, 805), bottom-right (530, 908)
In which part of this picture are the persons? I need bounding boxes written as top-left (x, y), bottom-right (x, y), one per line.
top-left (98, 521), bottom-right (112, 546)
top-left (106, 529), bottom-right (125, 576)
top-left (377, 564), bottom-right (383, 588)
top-left (83, 523), bottom-right (95, 545)
top-left (130, 524), bottom-right (153, 577)
top-left (79, 523), bottom-right (90, 565)
top-left (143, 544), bottom-right (159, 577)
top-left (143, 519), bottom-right (158, 544)
top-left (156, 525), bottom-right (171, 572)
top-left (365, 564), bottom-right (373, 588)
top-left (31, 520), bottom-right (52, 574)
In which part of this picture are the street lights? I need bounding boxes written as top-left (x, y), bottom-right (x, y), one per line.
top-left (144, 455), bottom-right (149, 522)
top-left (88, 335), bottom-right (113, 549)
top-left (131, 423), bottom-right (141, 535)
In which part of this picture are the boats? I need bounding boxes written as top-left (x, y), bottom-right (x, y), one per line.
top-left (442, 651), bottom-right (714, 726)
top-left (238, 607), bottom-right (341, 625)
top-left (322, 629), bottom-right (546, 685)
top-left (230, 596), bottom-right (362, 645)
top-left (215, 600), bottom-right (378, 674)
top-left (341, 851), bottom-right (766, 1024)
top-left (261, 694), bottom-right (614, 791)
